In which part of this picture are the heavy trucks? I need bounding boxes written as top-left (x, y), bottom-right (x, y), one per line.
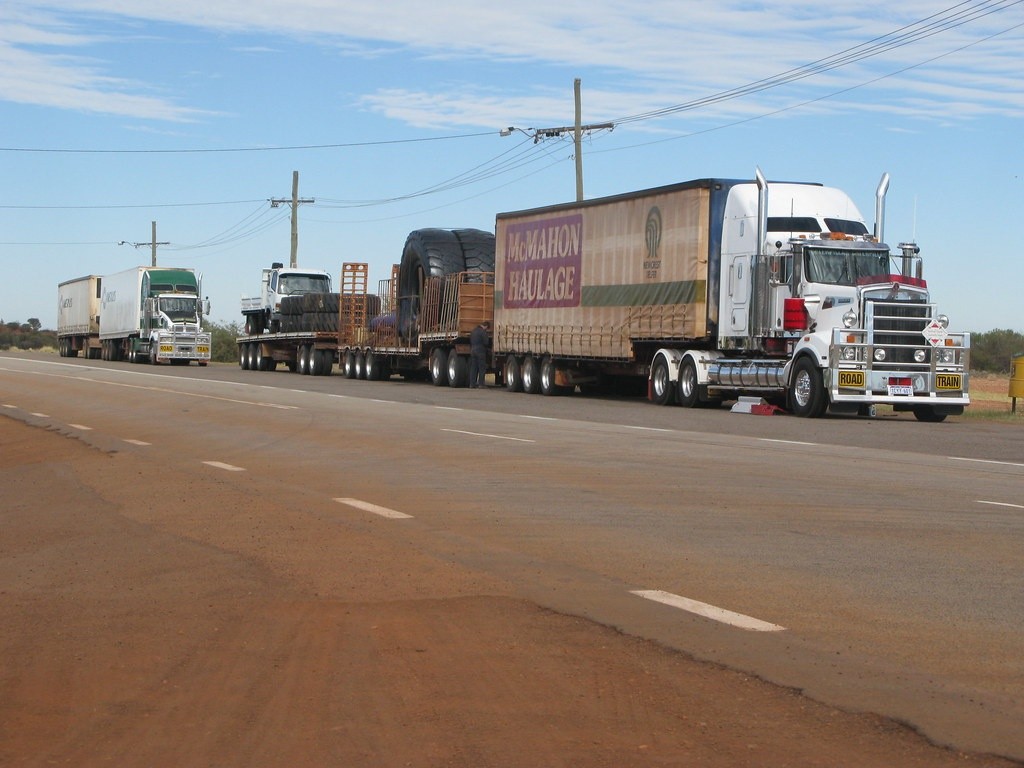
top-left (55, 265), bottom-right (214, 367)
top-left (231, 162), bottom-right (974, 423)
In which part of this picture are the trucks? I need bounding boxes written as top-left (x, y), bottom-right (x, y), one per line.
top-left (239, 260), bottom-right (333, 334)
